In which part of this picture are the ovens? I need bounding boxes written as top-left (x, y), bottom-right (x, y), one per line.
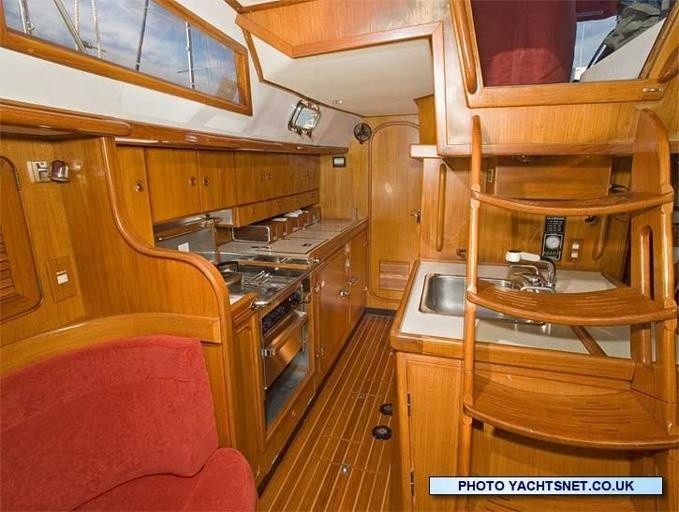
top-left (260, 286), bottom-right (308, 389)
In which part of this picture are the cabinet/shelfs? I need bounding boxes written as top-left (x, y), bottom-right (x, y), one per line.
top-left (315, 229), bottom-right (368, 388)
top-left (116, 146), bottom-right (319, 247)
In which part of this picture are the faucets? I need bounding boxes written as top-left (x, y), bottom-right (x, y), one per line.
top-left (507, 262), bottom-right (540, 278)
top-left (517, 285), bottom-right (556, 296)
top-left (503, 248), bottom-right (554, 284)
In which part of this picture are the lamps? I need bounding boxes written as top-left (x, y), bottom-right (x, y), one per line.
top-left (33, 160), bottom-right (71, 183)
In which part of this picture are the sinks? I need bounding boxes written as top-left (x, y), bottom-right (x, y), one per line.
top-left (417, 272), bottom-right (547, 325)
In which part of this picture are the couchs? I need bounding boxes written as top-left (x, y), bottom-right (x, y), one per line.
top-left (0, 335), bottom-right (259, 511)
